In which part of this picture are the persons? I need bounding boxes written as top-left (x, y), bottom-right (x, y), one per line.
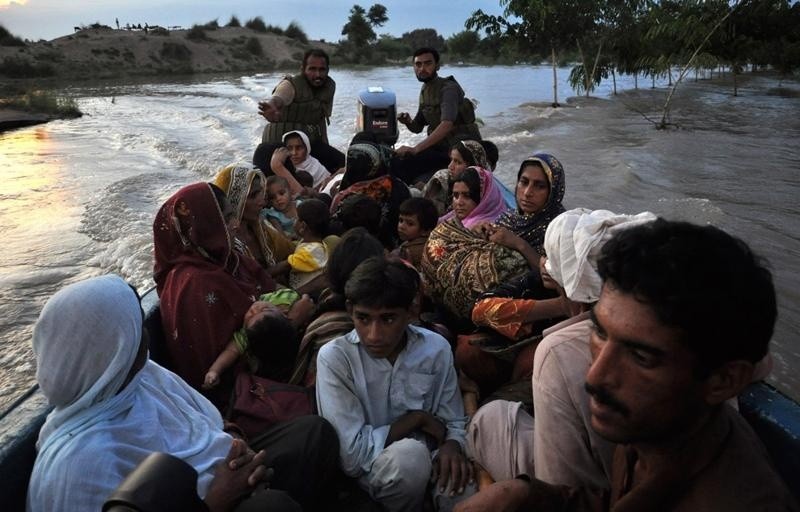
top-left (252, 47), bottom-right (346, 175)
top-left (397, 196), bottom-right (439, 269)
top-left (251, 130), bottom-right (331, 195)
top-left (451, 165), bottom-right (509, 231)
top-left (396, 46), bottom-right (498, 174)
top-left (25, 273), bottom-right (341, 511)
top-left (213, 161), bottom-right (341, 294)
top-left (203, 285), bottom-right (299, 390)
top-left (315, 255), bottom-right (476, 512)
top-left (410, 138), bottom-right (499, 215)
top-left (338, 193), bottom-right (382, 233)
top-left (463, 207), bottom-right (656, 491)
top-left (471, 152), bottom-right (565, 270)
top-left (289, 225), bottom-right (449, 391)
top-left (450, 219), bottom-right (800, 512)
top-left (267, 175), bottom-right (302, 242)
top-left (471, 249), bottom-right (566, 340)
top-left (152, 182), bottom-right (318, 419)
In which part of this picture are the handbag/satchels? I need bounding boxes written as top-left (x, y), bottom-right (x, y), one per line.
top-left (232, 371), bottom-right (315, 422)
